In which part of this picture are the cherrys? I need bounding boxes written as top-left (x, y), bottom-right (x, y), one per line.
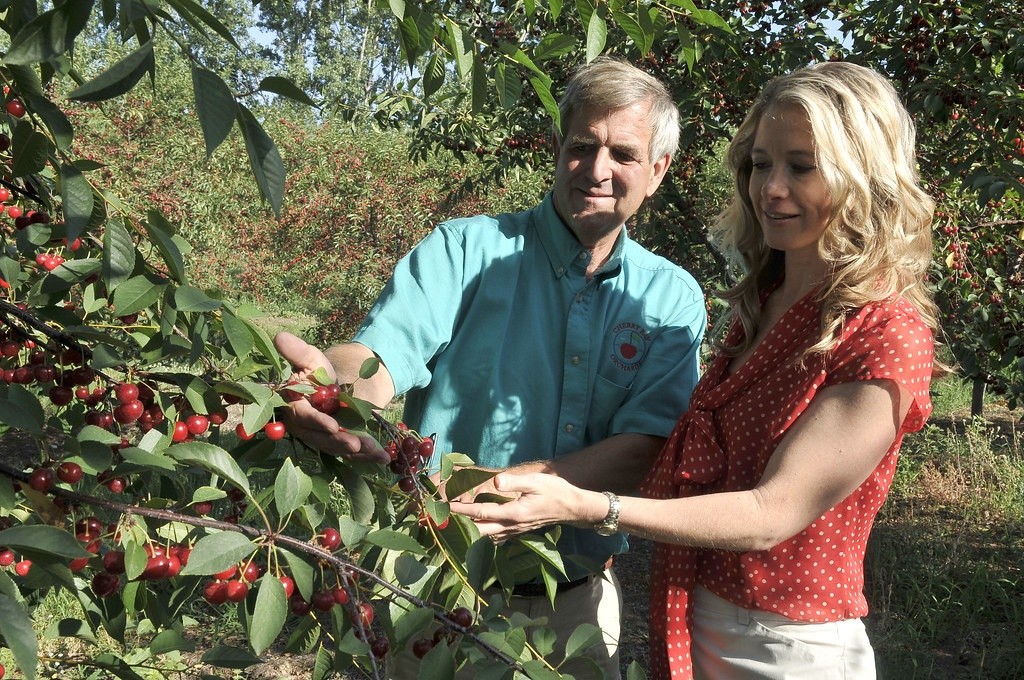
top-left (448, 0), bottom-right (1024, 317)
top-left (1, 74), bottom-right (479, 659)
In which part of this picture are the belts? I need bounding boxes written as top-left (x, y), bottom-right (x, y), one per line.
top-left (693, 586), bottom-right (794, 623)
top-left (489, 574), bottom-right (597, 597)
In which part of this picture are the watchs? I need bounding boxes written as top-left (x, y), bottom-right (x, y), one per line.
top-left (594, 491), bottom-right (621, 537)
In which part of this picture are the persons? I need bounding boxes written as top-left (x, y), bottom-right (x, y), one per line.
top-left (274, 59), bottom-right (708, 680)
top-left (449, 62), bottom-right (938, 680)
